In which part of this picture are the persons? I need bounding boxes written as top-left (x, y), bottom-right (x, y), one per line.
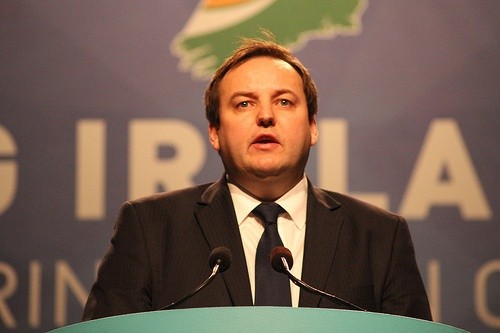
top-left (81, 38), bottom-right (434, 324)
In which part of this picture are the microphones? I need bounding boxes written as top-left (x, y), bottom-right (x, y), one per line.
top-left (271, 248), bottom-right (367, 311)
top-left (155, 246), bottom-right (232, 311)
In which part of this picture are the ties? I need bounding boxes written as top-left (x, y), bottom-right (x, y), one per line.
top-left (252, 202), bottom-right (292, 307)
